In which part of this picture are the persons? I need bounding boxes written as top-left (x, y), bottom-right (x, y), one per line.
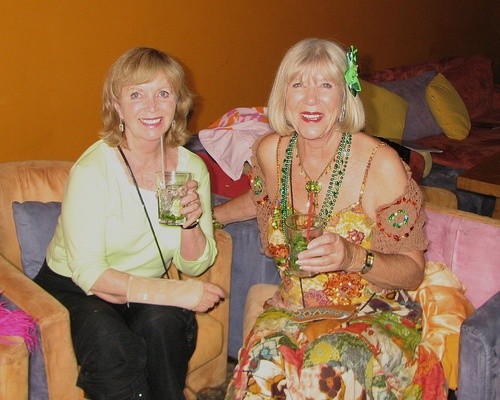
top-left (212, 38), bottom-right (448, 400)
top-left (33, 47), bottom-right (224, 400)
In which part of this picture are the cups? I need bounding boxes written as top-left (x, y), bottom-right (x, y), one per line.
top-left (156, 171), bottom-right (192, 226)
top-left (285, 214), bottom-right (323, 278)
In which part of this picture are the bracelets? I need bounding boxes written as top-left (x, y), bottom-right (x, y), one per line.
top-left (180, 221), bottom-right (199, 229)
top-left (211, 208), bottom-right (226, 228)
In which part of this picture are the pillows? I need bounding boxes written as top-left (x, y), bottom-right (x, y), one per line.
top-left (425, 72), bottom-right (472, 141)
top-left (445, 55), bottom-right (494, 119)
top-left (358, 78), bottom-right (410, 143)
top-left (377, 70), bottom-right (443, 141)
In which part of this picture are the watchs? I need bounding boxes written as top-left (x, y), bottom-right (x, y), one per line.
top-left (357, 249), bottom-right (374, 275)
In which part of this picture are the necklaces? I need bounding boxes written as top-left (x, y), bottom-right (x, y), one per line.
top-left (296, 138), bottom-right (341, 214)
top-left (114, 144), bottom-right (173, 279)
top-left (281, 130), bottom-right (352, 245)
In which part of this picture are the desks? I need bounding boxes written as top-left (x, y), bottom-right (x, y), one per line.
top-left (456, 151), bottom-right (500, 220)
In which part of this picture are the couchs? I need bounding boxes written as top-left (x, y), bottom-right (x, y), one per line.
top-left (0, 56), bottom-right (500, 400)
